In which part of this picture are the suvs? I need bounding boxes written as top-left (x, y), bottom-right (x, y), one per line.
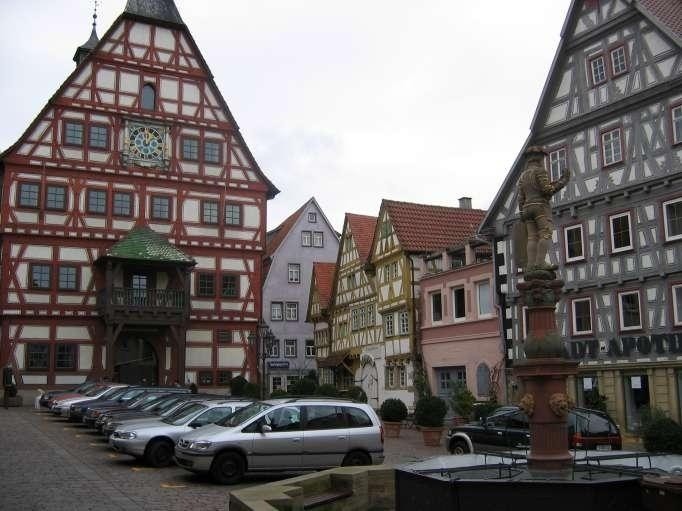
top-left (171, 398), bottom-right (386, 483)
top-left (443, 403), bottom-right (622, 462)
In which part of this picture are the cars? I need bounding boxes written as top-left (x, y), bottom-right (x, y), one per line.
top-left (108, 398), bottom-right (301, 470)
top-left (41, 383), bottom-right (228, 438)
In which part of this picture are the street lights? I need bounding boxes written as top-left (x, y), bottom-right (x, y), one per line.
top-left (248, 315), bottom-right (276, 397)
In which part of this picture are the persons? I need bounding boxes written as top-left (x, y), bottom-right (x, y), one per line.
top-left (517, 146), bottom-right (570, 273)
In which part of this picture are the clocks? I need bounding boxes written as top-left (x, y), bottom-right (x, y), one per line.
top-left (128, 120), bottom-right (166, 164)
